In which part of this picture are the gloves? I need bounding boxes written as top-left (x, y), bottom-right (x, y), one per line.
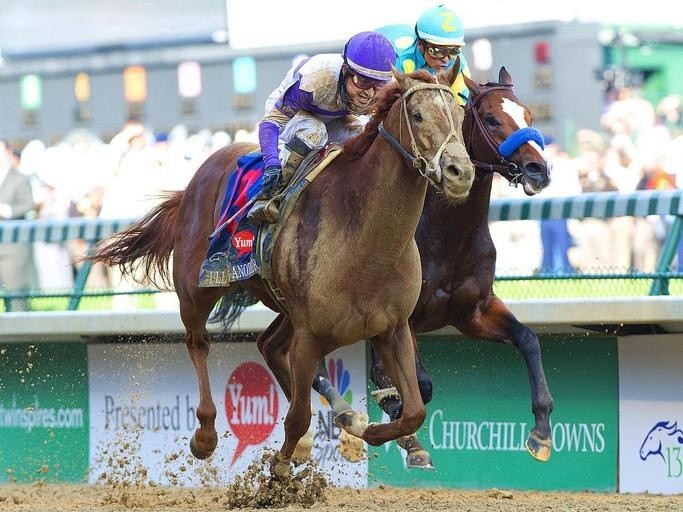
top-left (263, 166), bottom-right (282, 197)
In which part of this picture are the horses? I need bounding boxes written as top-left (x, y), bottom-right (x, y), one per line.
top-left (257, 65), bottom-right (553, 469)
top-left (65, 55), bottom-right (475, 484)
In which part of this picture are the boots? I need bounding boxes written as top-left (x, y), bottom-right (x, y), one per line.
top-left (247, 144), bottom-right (304, 226)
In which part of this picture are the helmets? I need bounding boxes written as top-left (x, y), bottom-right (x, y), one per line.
top-left (342, 31), bottom-right (397, 81)
top-left (415, 4), bottom-right (466, 47)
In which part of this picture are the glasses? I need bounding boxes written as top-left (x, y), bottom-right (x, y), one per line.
top-left (415, 26), bottom-right (461, 60)
top-left (344, 38), bottom-right (387, 91)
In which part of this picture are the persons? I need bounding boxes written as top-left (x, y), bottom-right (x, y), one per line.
top-left (1, 118), bottom-right (260, 314)
top-left (246, 31), bottom-right (397, 233)
top-left (397, 6), bottom-right (474, 113)
top-left (486, 104), bottom-right (682, 275)
